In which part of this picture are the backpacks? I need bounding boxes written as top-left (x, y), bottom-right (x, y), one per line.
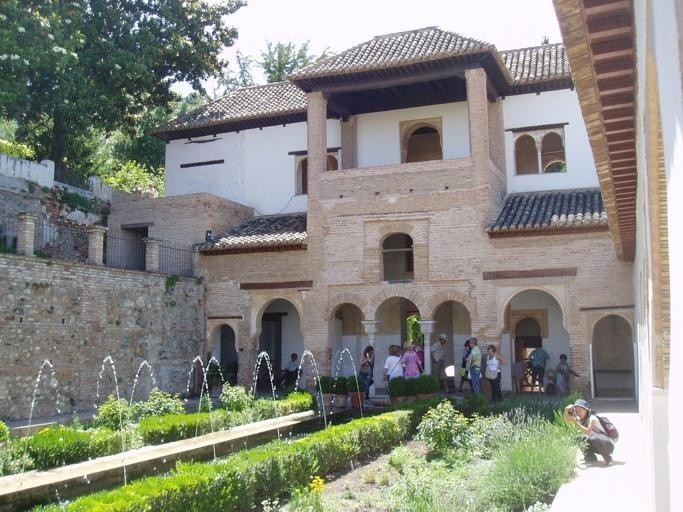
top-left (594, 415), bottom-right (619, 439)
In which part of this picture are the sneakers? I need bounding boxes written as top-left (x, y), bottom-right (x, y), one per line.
top-left (577, 459), bottom-right (614, 469)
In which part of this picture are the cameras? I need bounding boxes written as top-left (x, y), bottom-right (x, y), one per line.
top-left (568, 405), bottom-right (576, 416)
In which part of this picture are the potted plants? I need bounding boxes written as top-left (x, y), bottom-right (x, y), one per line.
top-left (388, 375), bottom-right (441, 405)
top-left (316, 375), bottom-right (367, 409)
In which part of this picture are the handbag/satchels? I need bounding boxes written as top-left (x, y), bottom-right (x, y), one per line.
top-left (485, 367), bottom-right (498, 381)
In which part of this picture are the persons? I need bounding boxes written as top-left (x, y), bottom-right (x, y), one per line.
top-left (358, 331), bottom-right (506, 404)
top-left (277, 353), bottom-right (301, 392)
top-left (555, 354), bottom-right (581, 397)
top-left (562, 399), bottom-right (619, 465)
top-left (530, 342), bottom-right (549, 393)
top-left (511, 359), bottom-right (533, 394)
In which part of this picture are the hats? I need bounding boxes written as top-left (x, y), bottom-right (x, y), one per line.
top-left (438, 334), bottom-right (448, 342)
top-left (573, 399), bottom-right (593, 411)
